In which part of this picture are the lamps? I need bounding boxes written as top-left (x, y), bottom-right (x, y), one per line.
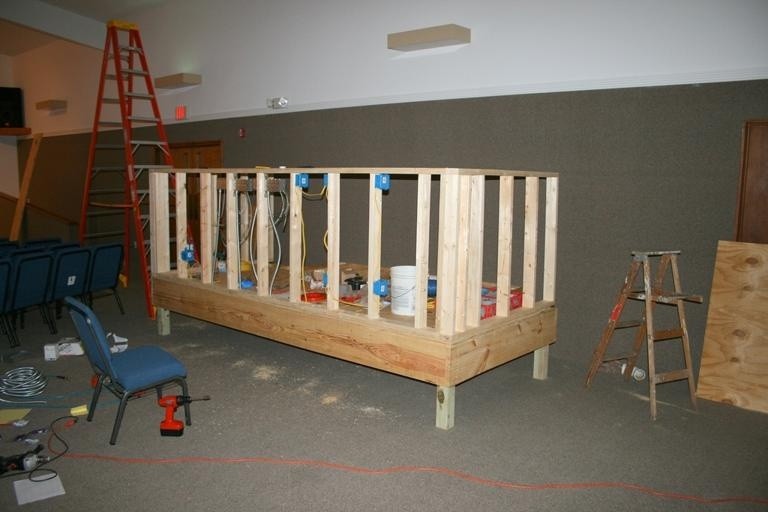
top-left (387, 24), bottom-right (472, 52)
top-left (153, 73), bottom-right (201, 90)
top-left (36, 99), bottom-right (66, 111)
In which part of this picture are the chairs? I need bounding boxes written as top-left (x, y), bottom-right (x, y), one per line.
top-left (63, 296), bottom-right (193, 447)
top-left (1, 237), bottom-right (126, 348)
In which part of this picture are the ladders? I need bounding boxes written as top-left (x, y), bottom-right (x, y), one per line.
top-left (79, 20), bottom-right (201, 319)
top-left (585, 251), bottom-right (704, 422)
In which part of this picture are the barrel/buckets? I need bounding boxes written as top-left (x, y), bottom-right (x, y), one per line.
top-left (390, 265), bottom-right (416, 316)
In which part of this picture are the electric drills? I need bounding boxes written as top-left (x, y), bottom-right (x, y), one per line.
top-left (159, 395), bottom-right (210, 436)
